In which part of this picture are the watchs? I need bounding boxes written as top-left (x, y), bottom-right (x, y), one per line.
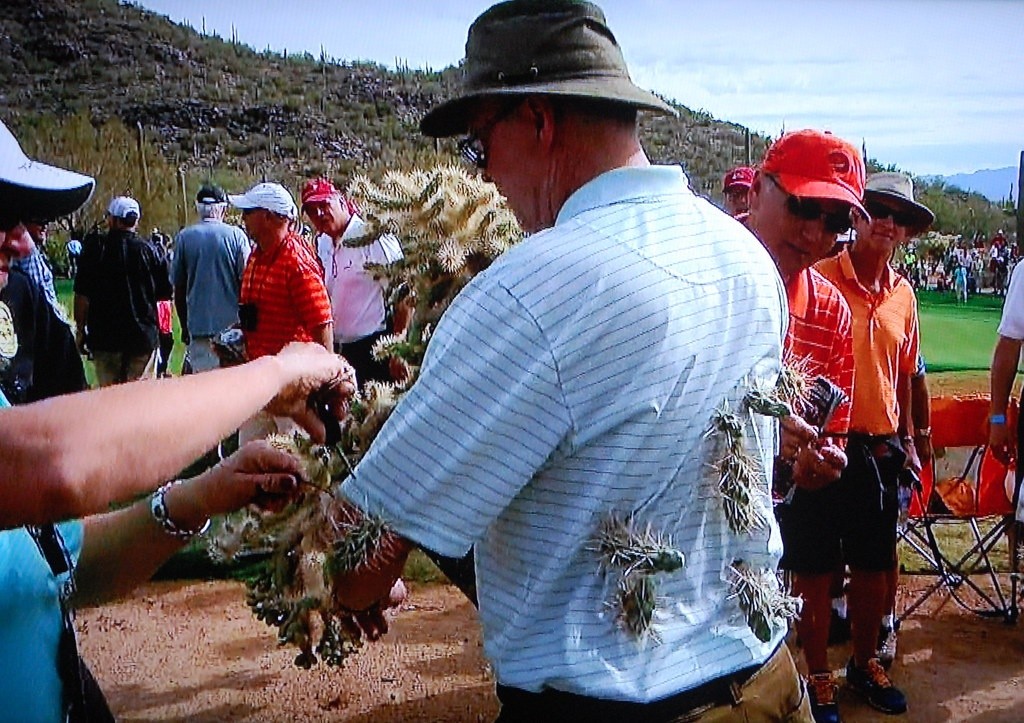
top-left (914, 426), bottom-right (932, 438)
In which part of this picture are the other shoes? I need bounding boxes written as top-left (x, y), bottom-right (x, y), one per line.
top-left (796, 609), bottom-right (849, 648)
top-left (875, 624), bottom-right (897, 671)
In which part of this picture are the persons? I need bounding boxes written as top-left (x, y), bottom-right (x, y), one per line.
top-left (315, 0), bottom-right (817, 723)
top-left (716, 131), bottom-right (1024, 723)
top-left (0, 120), bottom-right (411, 723)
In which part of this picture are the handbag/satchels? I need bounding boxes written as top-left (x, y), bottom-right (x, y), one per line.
top-left (57, 625), bottom-right (115, 723)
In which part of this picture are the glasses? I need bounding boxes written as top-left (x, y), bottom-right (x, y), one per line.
top-left (459, 101), bottom-right (516, 169)
top-left (866, 202), bottom-right (913, 227)
top-left (765, 174), bottom-right (853, 235)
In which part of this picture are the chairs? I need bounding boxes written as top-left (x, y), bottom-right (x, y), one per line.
top-left (893, 396), bottom-right (1019, 630)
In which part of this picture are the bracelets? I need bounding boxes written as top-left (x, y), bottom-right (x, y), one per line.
top-left (902, 436), bottom-right (913, 445)
top-left (990, 413), bottom-right (1006, 425)
top-left (149, 480), bottom-right (212, 540)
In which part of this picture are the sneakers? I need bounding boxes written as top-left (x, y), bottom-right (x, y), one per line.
top-left (806, 670), bottom-right (838, 723)
top-left (847, 655), bottom-right (907, 715)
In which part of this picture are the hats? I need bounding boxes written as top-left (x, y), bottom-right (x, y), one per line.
top-left (420, 0), bottom-right (679, 137)
top-left (864, 172), bottom-right (936, 238)
top-left (301, 180), bottom-right (340, 216)
top-left (721, 168), bottom-right (756, 193)
top-left (761, 129), bottom-right (871, 225)
top-left (108, 197), bottom-right (141, 219)
top-left (196, 186), bottom-right (227, 204)
top-left (228, 182), bottom-right (297, 220)
top-left (0, 119), bottom-right (97, 222)
